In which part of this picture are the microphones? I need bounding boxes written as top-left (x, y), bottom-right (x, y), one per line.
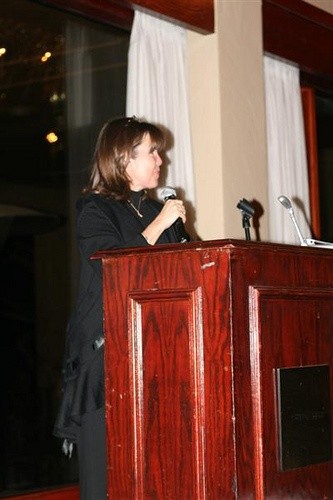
top-left (157, 186), bottom-right (189, 243)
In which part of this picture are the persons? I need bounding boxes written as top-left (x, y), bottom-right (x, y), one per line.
top-left (52, 117), bottom-right (191, 500)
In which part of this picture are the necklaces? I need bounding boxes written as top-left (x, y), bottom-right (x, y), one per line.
top-left (124, 195), bottom-right (143, 218)
